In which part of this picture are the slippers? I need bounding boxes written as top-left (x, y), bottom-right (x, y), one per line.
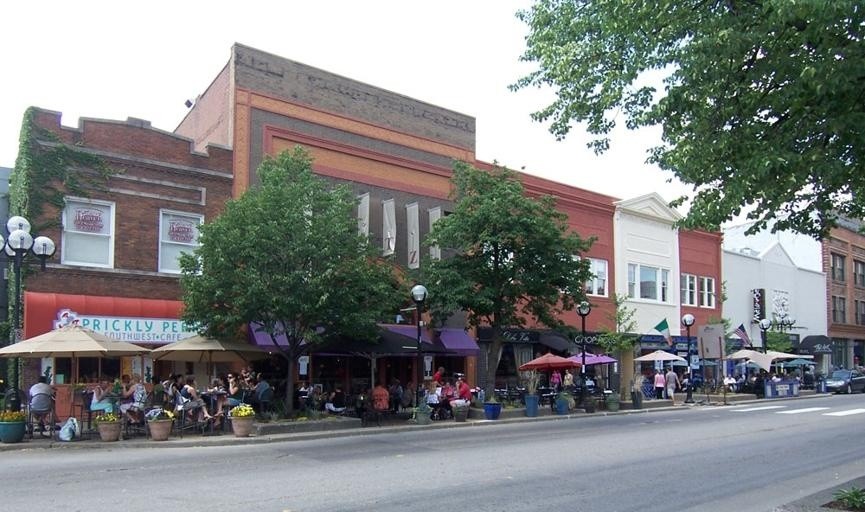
top-left (187, 412), bottom-right (224, 428)
top-left (128, 420), bottom-right (140, 426)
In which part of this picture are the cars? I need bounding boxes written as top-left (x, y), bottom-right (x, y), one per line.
top-left (820, 366), bottom-right (865, 397)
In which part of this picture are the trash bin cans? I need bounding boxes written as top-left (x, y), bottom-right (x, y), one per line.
top-left (524, 394), bottom-right (540, 417)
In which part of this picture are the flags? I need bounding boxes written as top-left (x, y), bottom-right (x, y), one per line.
top-left (654, 319), bottom-right (673, 346)
top-left (734, 323), bottom-right (751, 346)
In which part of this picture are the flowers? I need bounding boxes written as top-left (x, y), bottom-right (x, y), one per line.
top-left (0, 411), bottom-right (27, 423)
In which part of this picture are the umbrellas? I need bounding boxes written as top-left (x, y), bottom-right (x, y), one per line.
top-left (150, 333), bottom-right (267, 387)
top-left (717, 348), bottom-right (765, 372)
top-left (0, 322), bottom-right (150, 402)
top-left (634, 350), bottom-right (686, 372)
top-left (566, 351), bottom-right (618, 377)
top-left (737, 349), bottom-right (816, 372)
top-left (519, 352), bottom-right (581, 386)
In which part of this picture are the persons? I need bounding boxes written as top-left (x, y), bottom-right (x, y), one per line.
top-left (652, 365), bottom-right (817, 394)
top-left (292, 367), bottom-right (473, 419)
top-left (665, 367), bottom-right (681, 403)
top-left (550, 368), bottom-right (606, 393)
top-left (28, 376), bottom-right (51, 427)
top-left (47, 380), bottom-right (62, 423)
top-left (654, 369), bottom-right (665, 399)
top-left (90, 364), bottom-right (271, 431)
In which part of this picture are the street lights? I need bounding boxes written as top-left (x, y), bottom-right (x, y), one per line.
top-left (408, 283), bottom-right (430, 413)
top-left (576, 299), bottom-right (596, 411)
top-left (0, 214), bottom-right (61, 428)
top-left (758, 319), bottom-right (771, 354)
top-left (680, 312), bottom-right (700, 405)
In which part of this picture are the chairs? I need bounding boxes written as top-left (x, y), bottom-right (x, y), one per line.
top-left (3, 387), bottom-right (55, 439)
top-left (69, 385), bottom-right (276, 441)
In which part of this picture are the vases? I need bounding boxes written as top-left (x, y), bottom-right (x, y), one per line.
top-left (0, 422), bottom-right (26, 443)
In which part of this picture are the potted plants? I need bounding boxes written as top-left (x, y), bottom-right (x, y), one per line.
top-left (451, 367), bottom-right (621, 422)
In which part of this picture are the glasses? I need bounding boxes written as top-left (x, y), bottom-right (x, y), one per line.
top-left (458, 379), bottom-right (463, 381)
top-left (227, 377), bottom-right (233, 378)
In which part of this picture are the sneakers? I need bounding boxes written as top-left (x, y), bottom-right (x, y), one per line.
top-left (38, 421), bottom-right (47, 432)
